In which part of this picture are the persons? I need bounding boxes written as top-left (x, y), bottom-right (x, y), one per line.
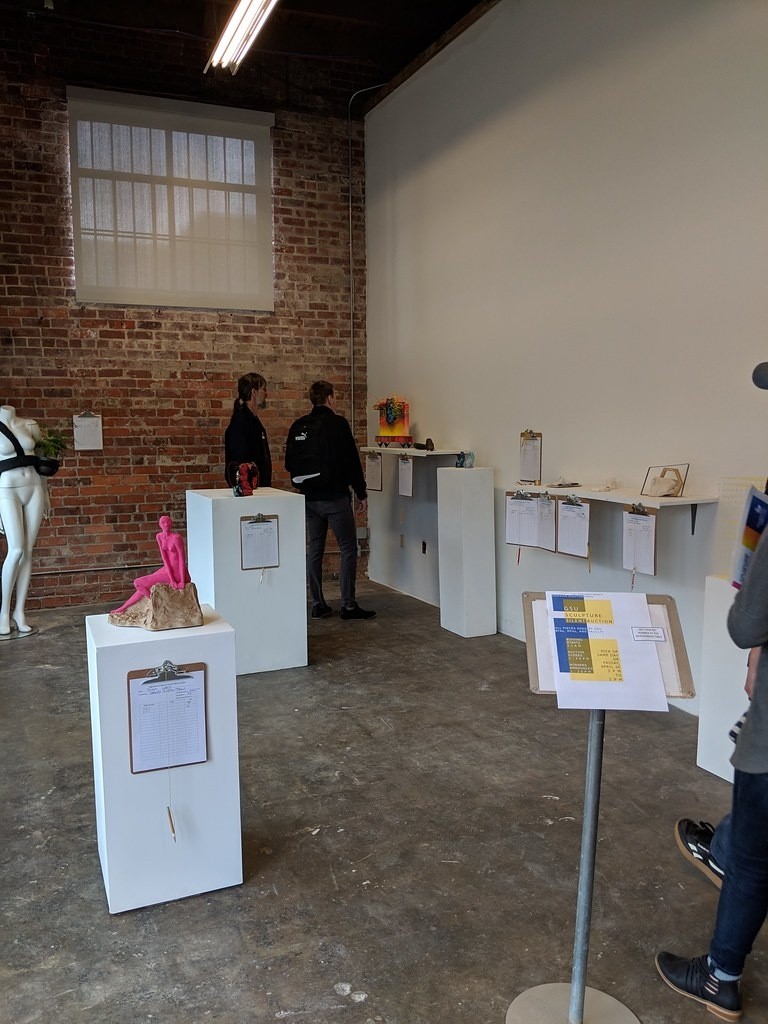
top-left (0, 405), bottom-right (50, 634)
top-left (111, 516), bottom-right (191, 613)
top-left (655, 362), bottom-right (768, 1023)
top-left (224, 373), bottom-right (272, 487)
top-left (284, 380), bottom-right (376, 620)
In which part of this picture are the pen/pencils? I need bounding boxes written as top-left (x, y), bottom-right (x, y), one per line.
top-left (631, 568), bottom-right (635, 590)
top-left (518, 548), bottom-right (520, 565)
top-left (166, 807), bottom-right (177, 843)
top-left (587, 543), bottom-right (591, 574)
top-left (260, 570), bottom-right (264, 583)
top-left (400, 508), bottom-right (403, 524)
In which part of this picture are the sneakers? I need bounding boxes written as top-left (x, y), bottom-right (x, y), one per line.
top-left (674, 817), bottom-right (725, 890)
top-left (339, 601), bottom-right (377, 621)
top-left (311, 605), bottom-right (333, 620)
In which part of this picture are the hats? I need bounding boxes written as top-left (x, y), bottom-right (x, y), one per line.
top-left (752, 361), bottom-right (768, 390)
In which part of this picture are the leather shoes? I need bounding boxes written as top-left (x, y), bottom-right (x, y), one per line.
top-left (654, 950), bottom-right (744, 1022)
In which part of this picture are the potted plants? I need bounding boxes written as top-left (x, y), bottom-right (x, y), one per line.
top-left (32, 421), bottom-right (69, 476)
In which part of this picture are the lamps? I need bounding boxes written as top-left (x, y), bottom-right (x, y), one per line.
top-left (204, 0), bottom-right (281, 74)
